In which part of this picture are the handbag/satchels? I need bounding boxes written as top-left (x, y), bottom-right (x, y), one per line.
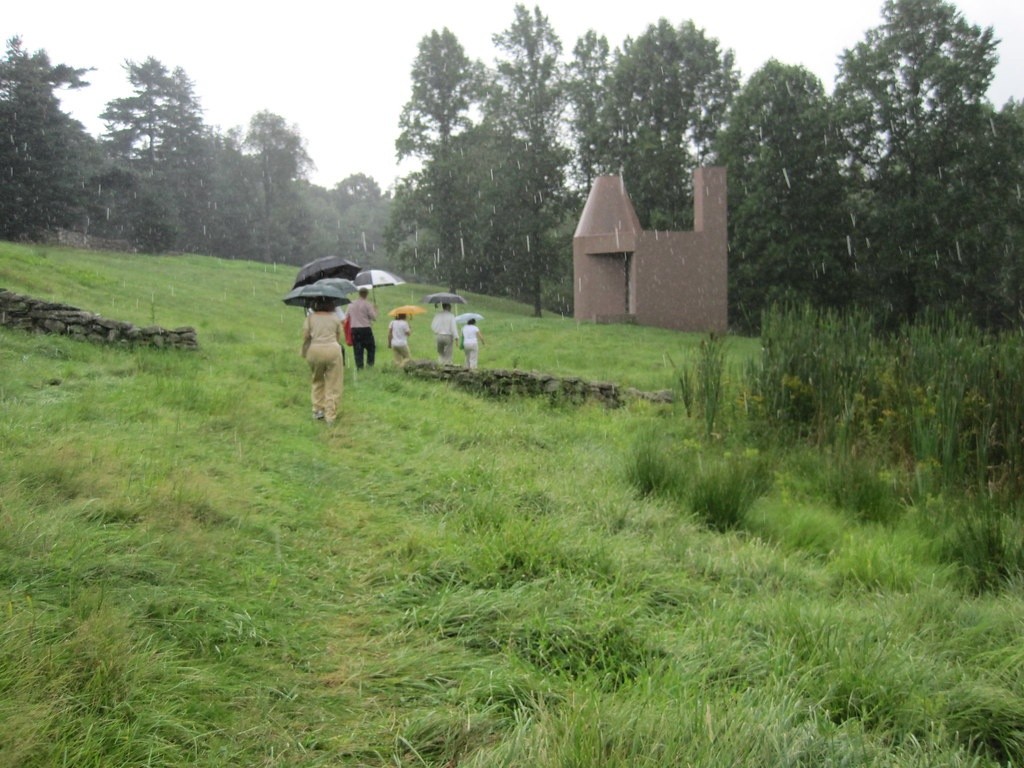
top-left (460, 330), bottom-right (464, 349)
top-left (344, 320), bottom-right (353, 346)
top-left (301, 337), bottom-right (312, 357)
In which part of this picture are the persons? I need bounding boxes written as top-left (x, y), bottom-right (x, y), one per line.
top-left (344, 287), bottom-right (377, 369)
top-left (388, 314), bottom-right (411, 367)
top-left (307, 307), bottom-right (345, 365)
top-left (431, 304), bottom-right (459, 363)
top-left (460, 319), bottom-right (485, 370)
top-left (301, 297), bottom-right (343, 421)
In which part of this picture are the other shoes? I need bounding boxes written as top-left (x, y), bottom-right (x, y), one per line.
top-left (312, 410), bottom-right (323, 419)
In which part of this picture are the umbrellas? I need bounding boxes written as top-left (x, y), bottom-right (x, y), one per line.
top-left (456, 313), bottom-right (484, 322)
top-left (389, 305), bottom-right (426, 317)
top-left (313, 277), bottom-right (358, 295)
top-left (282, 285), bottom-right (350, 307)
top-left (292, 255), bottom-right (362, 290)
top-left (420, 292), bottom-right (467, 303)
top-left (350, 269), bottom-right (405, 300)
